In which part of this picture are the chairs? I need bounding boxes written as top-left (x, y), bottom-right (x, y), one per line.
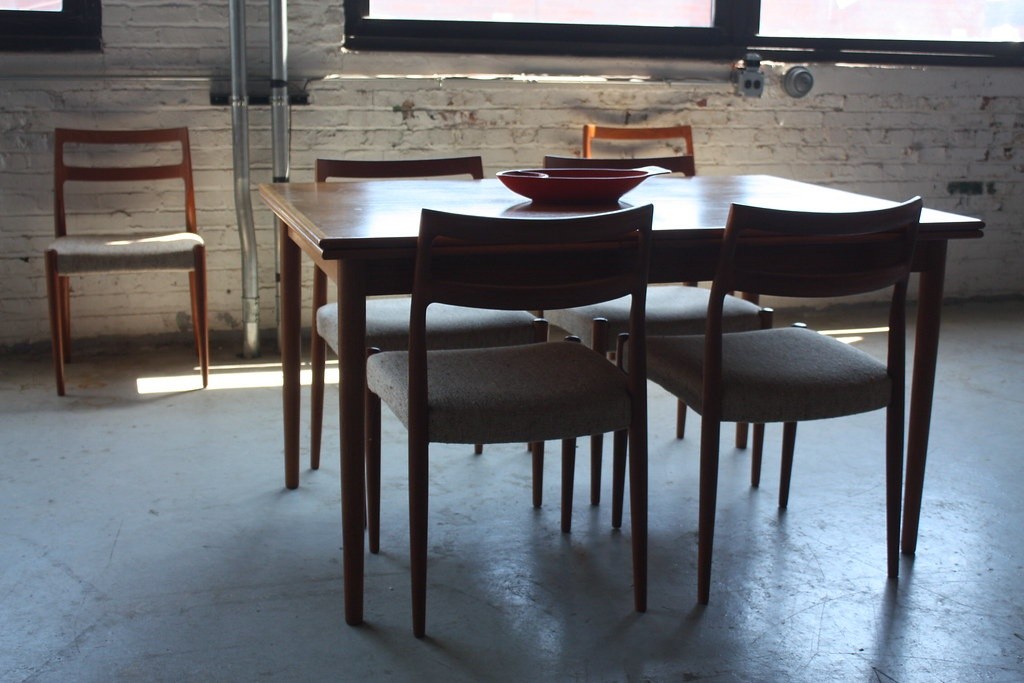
top-left (610, 195), bottom-right (924, 608)
top-left (579, 125), bottom-right (696, 178)
top-left (44, 127), bottom-right (210, 399)
top-left (310, 154), bottom-right (552, 505)
top-left (366, 201), bottom-right (656, 638)
top-left (543, 154), bottom-right (775, 507)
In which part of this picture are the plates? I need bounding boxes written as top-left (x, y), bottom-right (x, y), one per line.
top-left (497, 164), bottom-right (672, 202)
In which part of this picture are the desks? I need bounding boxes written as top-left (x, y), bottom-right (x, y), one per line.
top-left (260, 172), bottom-right (988, 629)
